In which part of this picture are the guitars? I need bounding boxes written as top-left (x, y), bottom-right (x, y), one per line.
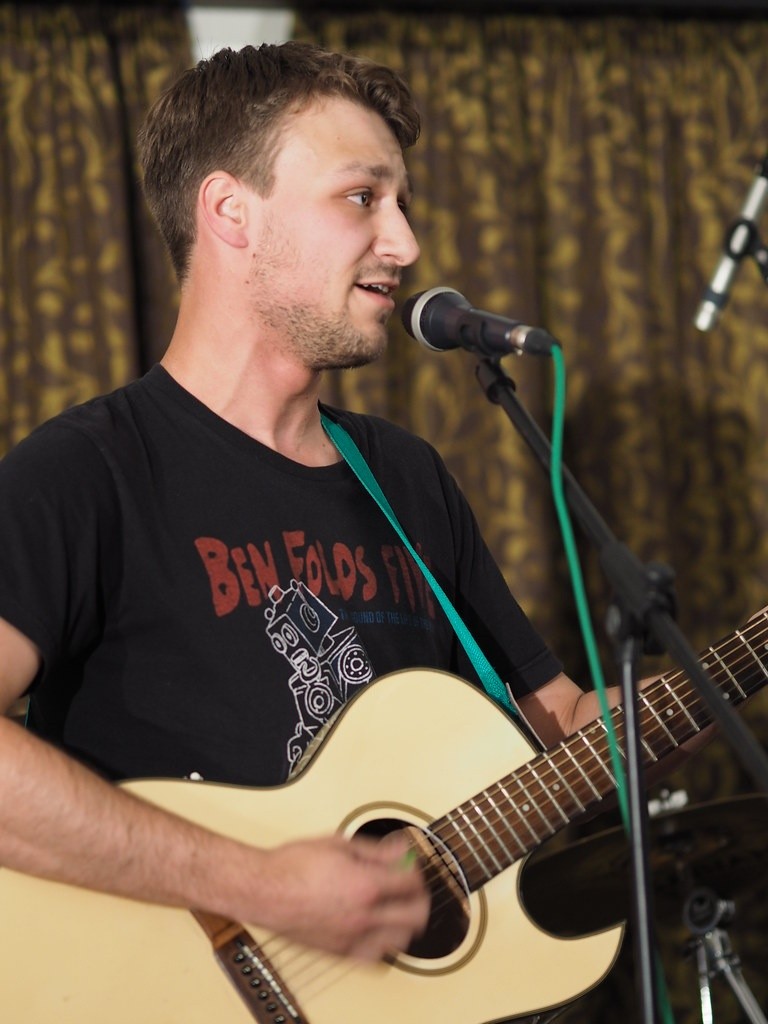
top-left (1, 606), bottom-right (768, 1023)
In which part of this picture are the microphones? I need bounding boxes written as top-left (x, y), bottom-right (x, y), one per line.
top-left (694, 149), bottom-right (768, 333)
top-left (402, 286), bottom-right (562, 359)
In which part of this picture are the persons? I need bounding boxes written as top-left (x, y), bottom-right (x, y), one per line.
top-left (0, 36), bottom-right (766, 966)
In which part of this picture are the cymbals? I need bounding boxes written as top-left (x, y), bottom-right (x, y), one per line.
top-left (520, 788), bottom-right (766, 915)
